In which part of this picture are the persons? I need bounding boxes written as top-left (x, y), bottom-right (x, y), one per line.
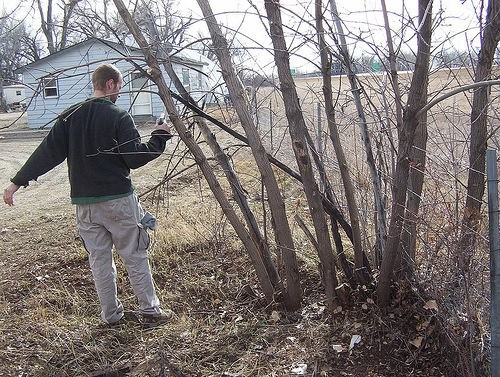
top-left (4, 64), bottom-right (172, 324)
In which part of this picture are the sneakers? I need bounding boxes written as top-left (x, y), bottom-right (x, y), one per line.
top-left (138, 307), bottom-right (174, 329)
